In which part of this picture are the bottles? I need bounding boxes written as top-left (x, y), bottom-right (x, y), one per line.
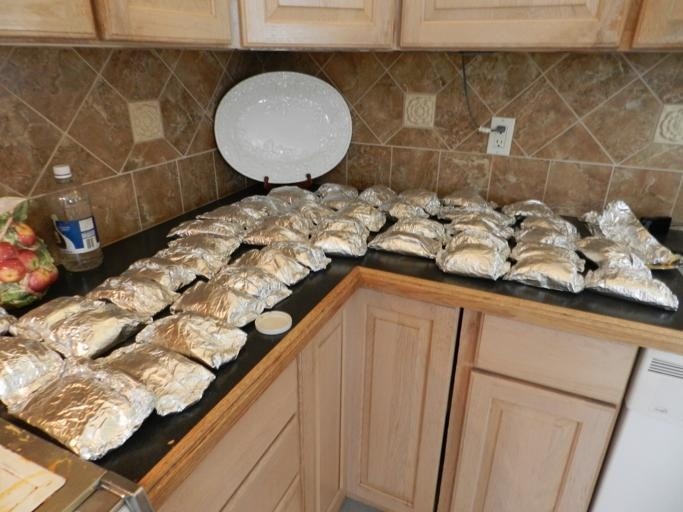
top-left (48, 164), bottom-right (100, 273)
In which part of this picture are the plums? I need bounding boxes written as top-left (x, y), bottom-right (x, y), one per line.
top-left (0, 222), bottom-right (59, 291)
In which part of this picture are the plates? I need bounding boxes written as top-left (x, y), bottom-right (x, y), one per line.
top-left (213, 71), bottom-right (352, 184)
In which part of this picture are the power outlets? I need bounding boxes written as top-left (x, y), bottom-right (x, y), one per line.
top-left (485, 117), bottom-right (516, 158)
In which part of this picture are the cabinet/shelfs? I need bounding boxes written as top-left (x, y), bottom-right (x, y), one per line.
top-left (448, 311), bottom-right (638, 512)
top-left (305, 290), bottom-right (459, 511)
top-left (155, 358), bottom-right (305, 512)
